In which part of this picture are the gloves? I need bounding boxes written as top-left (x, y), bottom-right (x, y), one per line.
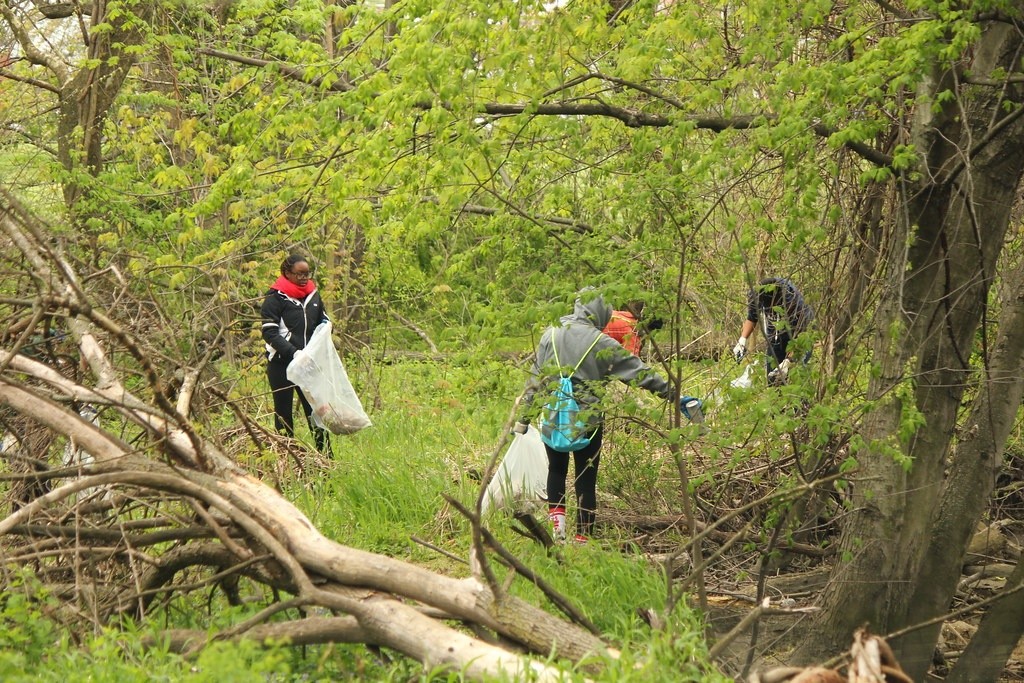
top-left (679, 395), bottom-right (703, 420)
top-left (513, 421), bottom-right (528, 435)
top-left (767, 359), bottom-right (791, 380)
top-left (733, 337), bottom-right (749, 365)
top-left (292, 350), bottom-right (317, 372)
top-left (322, 319), bottom-right (328, 323)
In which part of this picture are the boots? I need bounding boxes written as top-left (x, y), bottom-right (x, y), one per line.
top-left (576, 534), bottom-right (588, 544)
top-left (549, 508), bottom-right (566, 540)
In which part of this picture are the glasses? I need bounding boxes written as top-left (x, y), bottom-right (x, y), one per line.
top-left (283, 270), bottom-right (312, 280)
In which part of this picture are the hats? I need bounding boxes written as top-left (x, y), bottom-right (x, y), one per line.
top-left (636, 301), bottom-right (664, 330)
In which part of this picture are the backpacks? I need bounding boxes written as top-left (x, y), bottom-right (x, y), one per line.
top-left (541, 325), bottom-right (604, 452)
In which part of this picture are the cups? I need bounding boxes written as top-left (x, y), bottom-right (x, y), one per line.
top-left (686, 400), bottom-right (703, 423)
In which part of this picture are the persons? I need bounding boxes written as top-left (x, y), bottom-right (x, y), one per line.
top-left (261, 255), bottom-right (332, 462)
top-left (732, 278), bottom-right (815, 388)
top-left (512, 285), bottom-right (705, 547)
top-left (600, 299), bottom-right (663, 361)
